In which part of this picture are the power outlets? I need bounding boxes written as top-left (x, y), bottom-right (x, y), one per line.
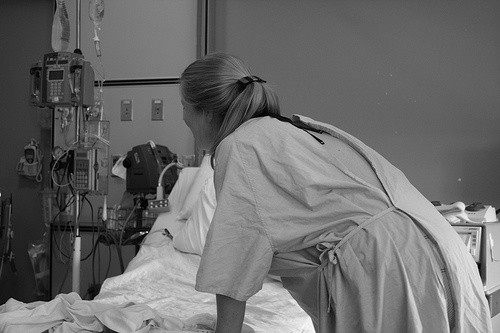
top-left (151, 99), bottom-right (164, 121)
top-left (121, 100), bottom-right (132, 121)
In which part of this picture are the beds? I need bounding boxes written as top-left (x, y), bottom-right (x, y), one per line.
top-left (0, 167), bottom-right (318, 333)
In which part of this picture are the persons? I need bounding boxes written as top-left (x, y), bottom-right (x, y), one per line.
top-left (180, 55), bottom-right (489, 333)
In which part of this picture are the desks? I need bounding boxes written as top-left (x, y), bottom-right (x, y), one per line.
top-left (50, 222), bottom-right (152, 301)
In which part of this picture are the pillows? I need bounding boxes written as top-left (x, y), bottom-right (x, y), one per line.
top-left (177, 153), bottom-right (216, 221)
top-left (172, 175), bottom-right (282, 282)
